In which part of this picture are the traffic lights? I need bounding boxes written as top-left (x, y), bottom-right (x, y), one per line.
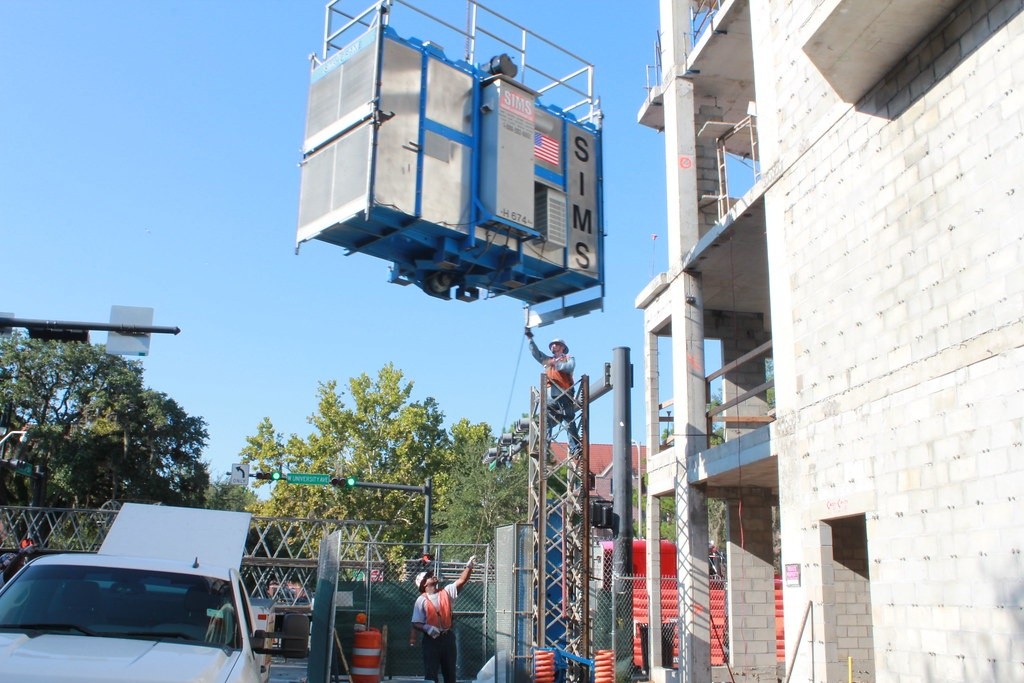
top-left (330, 476), bottom-right (357, 487)
top-left (256, 471), bottom-right (282, 480)
top-left (9, 460), bottom-right (27, 469)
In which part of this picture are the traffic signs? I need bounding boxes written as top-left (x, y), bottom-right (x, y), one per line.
top-left (231, 464), bottom-right (249, 485)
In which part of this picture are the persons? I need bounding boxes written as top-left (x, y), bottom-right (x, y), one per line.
top-left (524, 328), bottom-right (581, 465)
top-left (410, 555), bottom-right (476, 683)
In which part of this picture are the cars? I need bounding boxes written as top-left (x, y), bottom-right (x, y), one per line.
top-left (0, 502), bottom-right (275, 683)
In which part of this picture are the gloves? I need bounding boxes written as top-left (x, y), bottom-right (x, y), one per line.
top-left (427, 626), bottom-right (440, 639)
top-left (466, 554), bottom-right (477, 568)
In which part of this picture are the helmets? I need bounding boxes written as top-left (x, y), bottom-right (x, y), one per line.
top-left (415, 570), bottom-right (428, 589)
top-left (548, 339), bottom-right (569, 355)
